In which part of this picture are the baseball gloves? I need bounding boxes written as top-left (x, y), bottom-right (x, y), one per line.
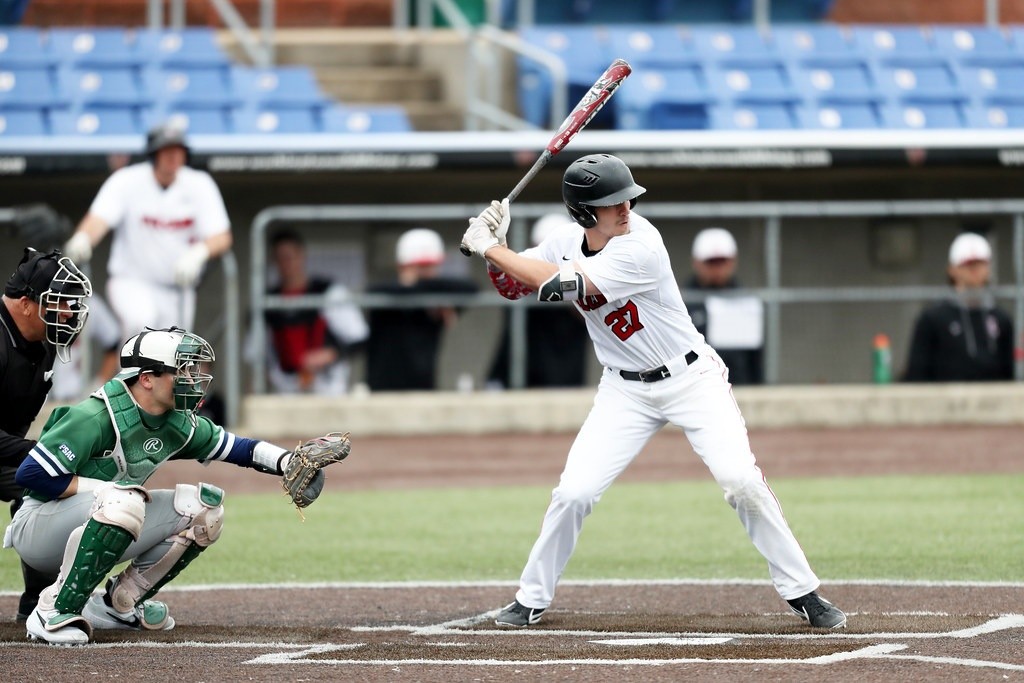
top-left (280, 435), bottom-right (352, 509)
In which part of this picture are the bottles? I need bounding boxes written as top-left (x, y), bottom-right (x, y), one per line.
top-left (873, 335), bottom-right (891, 384)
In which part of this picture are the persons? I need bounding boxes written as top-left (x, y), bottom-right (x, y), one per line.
top-left (244, 232), bottom-right (368, 395)
top-left (681, 228), bottom-right (764, 384)
top-left (3, 325), bottom-right (351, 648)
top-left (461, 153), bottom-right (848, 630)
top-left (898, 232), bottom-right (1015, 382)
top-left (485, 215), bottom-right (590, 389)
top-left (69, 126), bottom-right (232, 347)
top-left (0, 248), bottom-right (92, 622)
top-left (43, 295), bottom-right (119, 401)
top-left (348, 230), bottom-right (480, 391)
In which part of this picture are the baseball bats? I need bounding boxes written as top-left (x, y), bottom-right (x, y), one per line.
top-left (458, 57), bottom-right (634, 257)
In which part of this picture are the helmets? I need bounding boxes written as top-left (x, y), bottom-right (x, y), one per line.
top-left (114, 326), bottom-right (198, 380)
top-left (562, 154), bottom-right (646, 228)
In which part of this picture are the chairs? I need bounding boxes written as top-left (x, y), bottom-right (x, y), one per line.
top-left (0, 24), bottom-right (417, 140)
top-left (500, 24), bottom-right (1024, 133)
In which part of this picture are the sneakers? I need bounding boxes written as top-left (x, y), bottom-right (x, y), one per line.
top-left (26, 606), bottom-right (88, 648)
top-left (82, 591), bottom-right (175, 632)
top-left (493, 601), bottom-right (547, 626)
top-left (786, 590), bottom-right (847, 630)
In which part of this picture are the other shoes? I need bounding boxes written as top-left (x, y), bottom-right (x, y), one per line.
top-left (15, 592), bottom-right (39, 619)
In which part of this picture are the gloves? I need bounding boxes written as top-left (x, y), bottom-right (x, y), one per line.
top-left (477, 198), bottom-right (511, 245)
top-left (459, 216), bottom-right (499, 261)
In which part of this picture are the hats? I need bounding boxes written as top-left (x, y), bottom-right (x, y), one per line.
top-left (396, 228), bottom-right (447, 271)
top-left (692, 226), bottom-right (737, 263)
top-left (946, 232), bottom-right (991, 267)
top-left (3, 245), bottom-right (86, 304)
top-left (142, 125), bottom-right (193, 157)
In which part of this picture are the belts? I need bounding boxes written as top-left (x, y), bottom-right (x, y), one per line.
top-left (609, 351), bottom-right (698, 384)
